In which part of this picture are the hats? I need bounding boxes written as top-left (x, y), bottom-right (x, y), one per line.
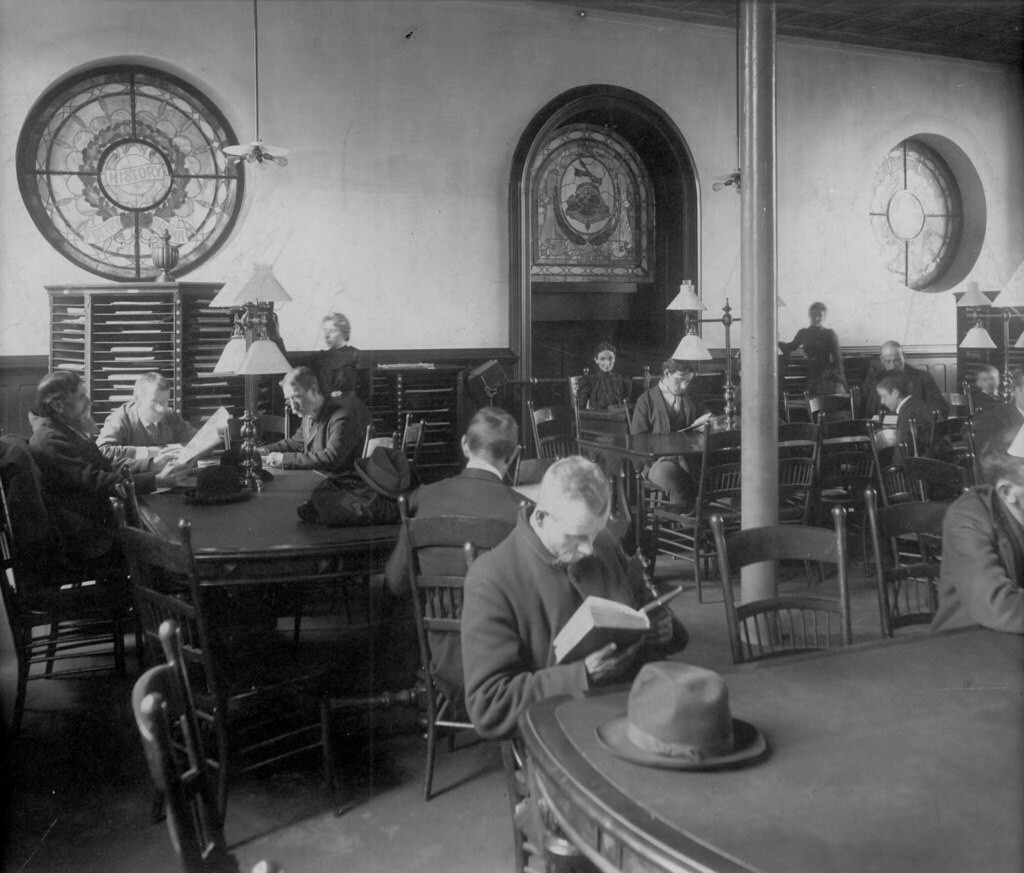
top-left (185, 464), bottom-right (250, 502)
top-left (595, 660), bottom-right (767, 767)
top-left (221, 448), bottom-right (274, 482)
top-left (355, 446), bottom-right (422, 498)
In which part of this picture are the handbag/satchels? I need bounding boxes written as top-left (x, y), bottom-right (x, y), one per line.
top-left (297, 470), bottom-right (400, 526)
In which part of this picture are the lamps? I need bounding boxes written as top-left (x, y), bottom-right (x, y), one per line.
top-left (221, 0), bottom-right (289, 167)
top-left (666, 278), bottom-right (741, 431)
top-left (957, 262), bottom-right (1024, 402)
top-left (208, 263), bottom-right (294, 490)
top-left (710, 0), bottom-right (742, 194)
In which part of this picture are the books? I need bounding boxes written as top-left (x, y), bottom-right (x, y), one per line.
top-left (155, 404), bottom-right (229, 479)
top-left (553, 584), bottom-right (683, 664)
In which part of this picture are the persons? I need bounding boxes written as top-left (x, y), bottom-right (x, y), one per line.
top-left (970, 364), bottom-right (1024, 468)
top-left (575, 343), bottom-right (633, 410)
top-left (29, 366), bottom-right (187, 637)
top-left (291, 311), bottom-right (371, 401)
top-left (875, 370), bottom-right (963, 500)
top-left (95, 373), bottom-right (198, 460)
top-left (779, 302), bottom-right (848, 399)
top-left (460, 456), bottom-right (689, 740)
top-left (929, 424), bottom-right (1024, 638)
top-left (848, 340), bottom-right (951, 420)
top-left (384, 406), bottom-right (536, 700)
top-left (252, 367), bottom-right (377, 474)
top-left (630, 357), bottom-right (717, 513)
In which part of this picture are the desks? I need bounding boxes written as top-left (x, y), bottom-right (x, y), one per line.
top-left (518, 624), bottom-right (1024, 872)
top-left (578, 405), bottom-right (634, 503)
top-left (134, 468), bottom-right (404, 781)
top-left (577, 432), bottom-right (874, 579)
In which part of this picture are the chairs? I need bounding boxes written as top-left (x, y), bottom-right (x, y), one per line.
top-left (0, 378), bottom-right (1023, 873)
